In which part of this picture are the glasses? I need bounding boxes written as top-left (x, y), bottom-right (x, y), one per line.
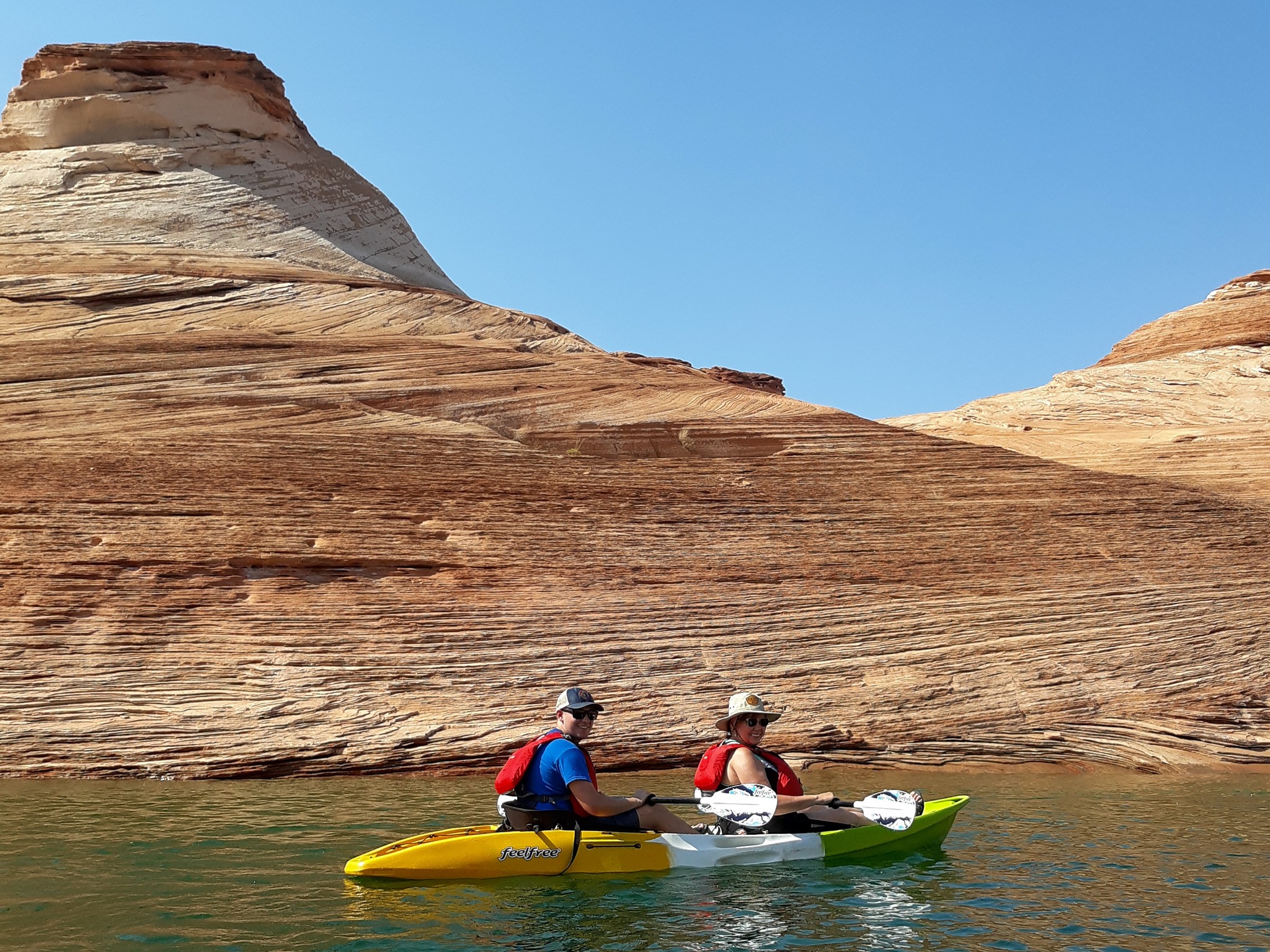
top-left (737, 718), bottom-right (769, 727)
top-left (561, 706), bottom-right (598, 720)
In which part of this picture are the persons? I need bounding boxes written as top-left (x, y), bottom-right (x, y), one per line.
top-left (495, 687), bottom-right (708, 835)
top-left (694, 692), bottom-right (924, 835)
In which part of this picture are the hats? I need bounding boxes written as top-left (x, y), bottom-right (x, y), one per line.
top-left (715, 692), bottom-right (781, 731)
top-left (556, 687), bottom-right (604, 713)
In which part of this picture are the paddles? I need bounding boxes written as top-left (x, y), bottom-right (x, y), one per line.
top-left (694, 786), bottom-right (917, 830)
top-left (496, 783), bottom-right (778, 827)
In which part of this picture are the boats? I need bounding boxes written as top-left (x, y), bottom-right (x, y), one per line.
top-left (344, 795), bottom-right (971, 879)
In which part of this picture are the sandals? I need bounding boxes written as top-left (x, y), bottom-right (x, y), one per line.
top-left (691, 824), bottom-right (708, 834)
top-left (909, 790), bottom-right (924, 817)
top-left (733, 828), bottom-right (747, 835)
